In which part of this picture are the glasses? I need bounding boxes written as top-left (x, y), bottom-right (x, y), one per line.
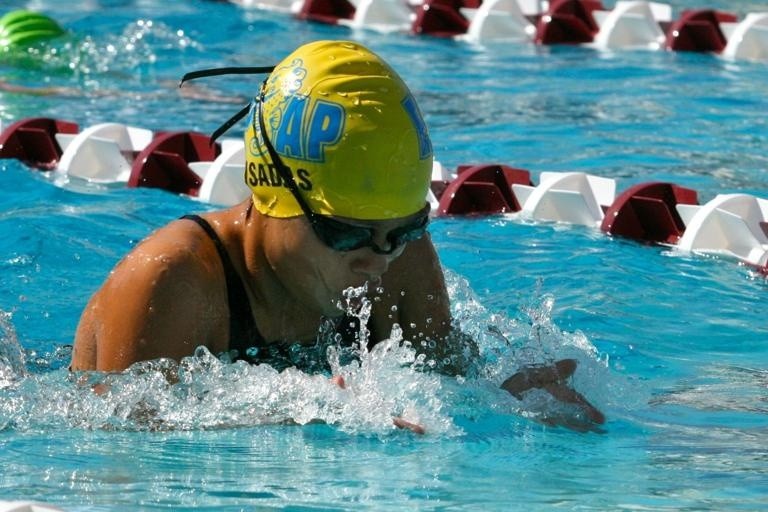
top-left (258, 77), bottom-right (430, 255)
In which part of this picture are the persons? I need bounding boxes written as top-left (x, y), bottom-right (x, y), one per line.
top-left (69, 39), bottom-right (610, 440)
